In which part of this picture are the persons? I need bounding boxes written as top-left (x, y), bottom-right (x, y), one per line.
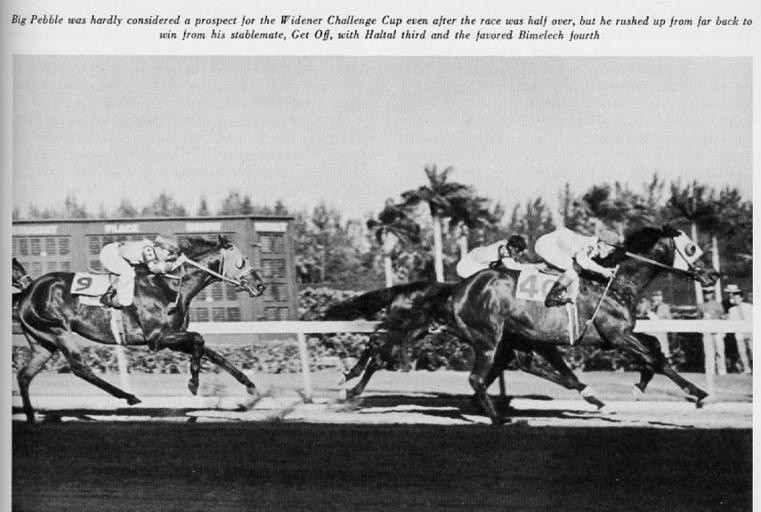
top-left (532, 215), bottom-right (628, 307)
top-left (638, 279), bottom-right (753, 374)
top-left (455, 235), bottom-right (525, 279)
top-left (98, 234), bottom-right (183, 308)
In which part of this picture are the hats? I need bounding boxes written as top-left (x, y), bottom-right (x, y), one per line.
top-left (702, 284), bottom-right (742, 293)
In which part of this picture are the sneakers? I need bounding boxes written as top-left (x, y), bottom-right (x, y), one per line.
top-left (99, 292), bottom-right (124, 310)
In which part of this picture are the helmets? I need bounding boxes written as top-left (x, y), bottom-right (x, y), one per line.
top-left (154, 235), bottom-right (180, 253)
top-left (599, 230), bottom-right (619, 247)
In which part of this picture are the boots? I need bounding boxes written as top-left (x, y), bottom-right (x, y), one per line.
top-left (544, 281), bottom-right (574, 308)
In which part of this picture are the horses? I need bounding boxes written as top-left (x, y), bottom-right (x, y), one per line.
top-left (320, 277), bottom-right (618, 416)
top-left (373, 222), bottom-right (720, 427)
top-left (12, 233), bottom-right (267, 420)
